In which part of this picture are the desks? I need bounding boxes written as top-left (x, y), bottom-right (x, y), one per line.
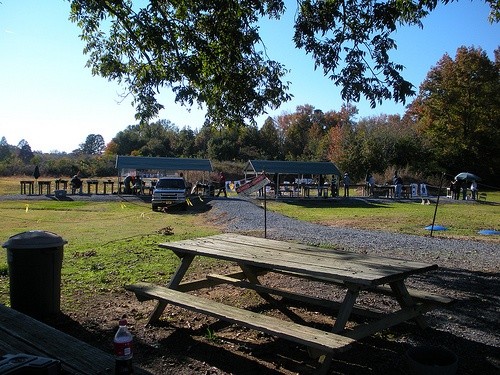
top-left (146, 233), bottom-right (438, 375)
top-left (0, 305), bottom-right (155, 375)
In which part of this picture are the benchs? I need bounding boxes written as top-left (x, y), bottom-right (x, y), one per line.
top-left (128, 282), bottom-right (356, 356)
top-left (274, 265), bottom-right (456, 341)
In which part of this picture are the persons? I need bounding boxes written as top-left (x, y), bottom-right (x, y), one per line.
top-left (367, 172), bottom-right (404, 199)
top-left (124, 175), bottom-right (206, 194)
top-left (70, 171), bottom-right (84, 195)
top-left (316, 172), bottom-right (350, 198)
top-left (216, 171), bottom-right (228, 198)
top-left (450, 179), bottom-right (477, 200)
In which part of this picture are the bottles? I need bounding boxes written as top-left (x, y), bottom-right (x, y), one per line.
top-left (113, 319), bottom-right (133, 375)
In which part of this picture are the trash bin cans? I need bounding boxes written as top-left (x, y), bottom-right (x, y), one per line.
top-left (2, 231), bottom-right (67, 321)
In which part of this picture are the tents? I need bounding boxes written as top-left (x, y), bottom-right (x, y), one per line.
top-left (115, 154), bottom-right (215, 195)
top-left (242, 160), bottom-right (343, 198)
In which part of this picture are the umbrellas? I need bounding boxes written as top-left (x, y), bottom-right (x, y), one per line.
top-left (454, 172), bottom-right (480, 181)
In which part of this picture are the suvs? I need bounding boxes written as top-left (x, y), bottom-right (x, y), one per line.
top-left (149, 176), bottom-right (192, 211)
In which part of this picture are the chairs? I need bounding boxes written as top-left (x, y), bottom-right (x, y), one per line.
top-left (479, 193), bottom-right (487, 201)
